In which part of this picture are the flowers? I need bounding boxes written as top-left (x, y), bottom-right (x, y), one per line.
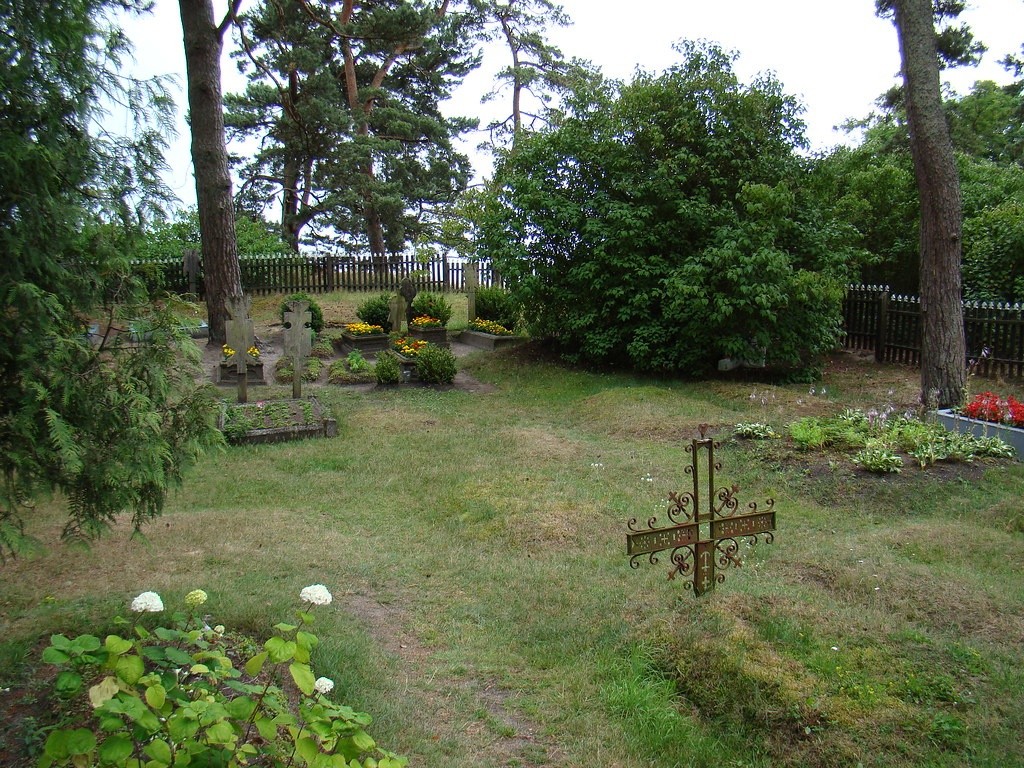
top-left (468, 316), bottom-right (514, 336)
top-left (345, 320), bottom-right (384, 336)
top-left (222, 342), bottom-right (260, 360)
top-left (314, 676), bottom-right (333, 694)
top-left (299, 583), bottom-right (332, 605)
top-left (960, 390), bottom-right (1024, 430)
top-left (130, 590), bottom-right (164, 612)
top-left (184, 589), bottom-right (208, 608)
top-left (411, 313), bottom-right (442, 327)
top-left (390, 333), bottom-right (431, 358)
